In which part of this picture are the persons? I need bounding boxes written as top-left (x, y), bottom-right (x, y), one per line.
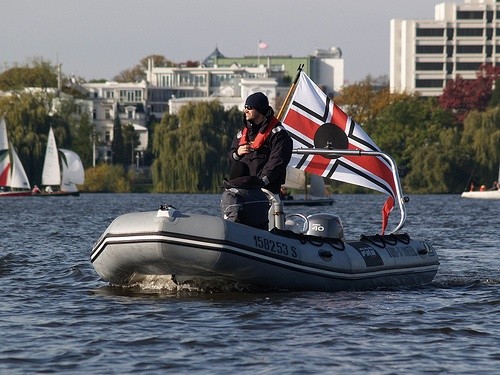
top-left (489, 180), bottom-right (500, 191)
top-left (221, 162), bottom-right (272, 230)
top-left (228, 91), bottom-right (293, 228)
top-left (32, 183), bottom-right (53, 193)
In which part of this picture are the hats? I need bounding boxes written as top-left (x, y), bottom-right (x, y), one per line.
top-left (229, 162), bottom-right (250, 179)
top-left (245, 92), bottom-right (269, 116)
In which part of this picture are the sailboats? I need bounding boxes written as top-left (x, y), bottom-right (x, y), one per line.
top-left (0, 116), bottom-right (35, 197)
top-left (32, 126), bottom-right (84, 196)
top-left (280, 164), bottom-right (335, 206)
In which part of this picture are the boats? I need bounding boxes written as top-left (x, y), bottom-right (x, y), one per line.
top-left (461, 183), bottom-right (500, 200)
top-left (90, 64), bottom-right (441, 291)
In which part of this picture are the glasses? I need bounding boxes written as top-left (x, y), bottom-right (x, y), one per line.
top-left (244, 106), bottom-right (253, 110)
